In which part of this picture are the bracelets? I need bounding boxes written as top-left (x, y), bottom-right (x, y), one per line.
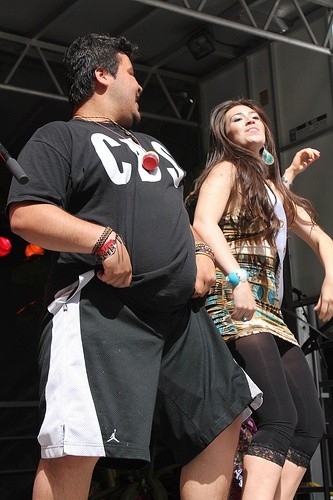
top-left (193, 243), bottom-right (216, 263)
top-left (92, 227), bottom-right (125, 260)
top-left (224, 267), bottom-right (250, 286)
top-left (281, 177), bottom-right (293, 187)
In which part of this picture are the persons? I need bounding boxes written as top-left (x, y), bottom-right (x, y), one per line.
top-left (281, 147), bottom-right (321, 188)
top-left (0, 33), bottom-right (264, 500)
top-left (184, 98), bottom-right (333, 500)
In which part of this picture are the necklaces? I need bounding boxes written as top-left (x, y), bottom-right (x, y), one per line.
top-left (73, 113), bottom-right (160, 171)
